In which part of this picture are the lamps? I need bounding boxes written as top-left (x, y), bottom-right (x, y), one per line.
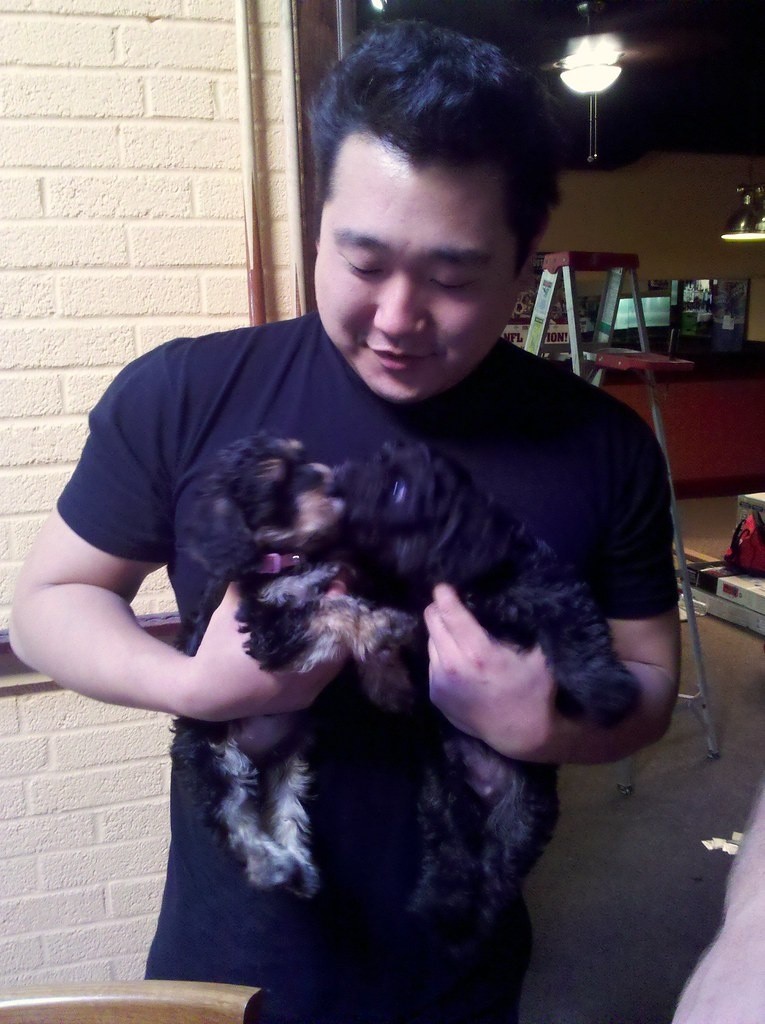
top-left (551, 0), bottom-right (625, 166)
top-left (718, 157), bottom-right (765, 244)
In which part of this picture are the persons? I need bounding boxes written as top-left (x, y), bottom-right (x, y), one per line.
top-left (8, 23), bottom-right (679, 1022)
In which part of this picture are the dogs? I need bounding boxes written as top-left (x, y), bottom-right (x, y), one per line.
top-left (322, 440), bottom-right (643, 929)
top-left (168, 432), bottom-right (417, 900)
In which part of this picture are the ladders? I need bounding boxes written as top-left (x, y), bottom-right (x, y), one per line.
top-left (523, 248), bottom-right (720, 799)
